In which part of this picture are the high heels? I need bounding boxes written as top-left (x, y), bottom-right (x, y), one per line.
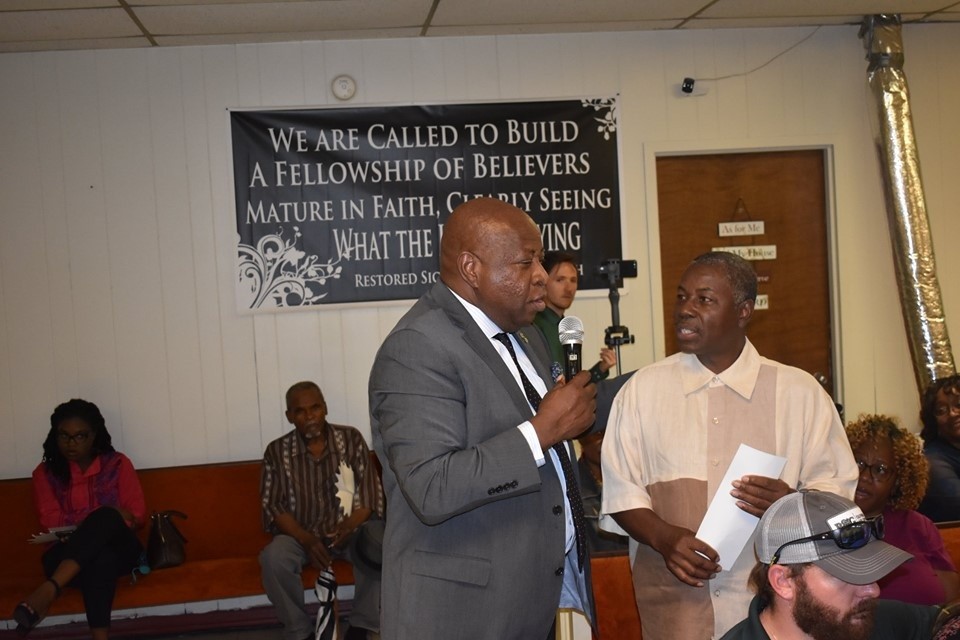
top-left (13, 578), bottom-right (62, 639)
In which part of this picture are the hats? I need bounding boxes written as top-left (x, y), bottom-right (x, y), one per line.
top-left (753, 488), bottom-right (916, 586)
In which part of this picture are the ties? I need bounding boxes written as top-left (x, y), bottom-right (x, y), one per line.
top-left (493, 332), bottom-right (587, 574)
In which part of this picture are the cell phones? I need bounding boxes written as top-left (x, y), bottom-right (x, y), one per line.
top-left (55, 529), bottom-right (75, 540)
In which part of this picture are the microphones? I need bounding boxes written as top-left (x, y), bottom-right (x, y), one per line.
top-left (558, 317), bottom-right (583, 384)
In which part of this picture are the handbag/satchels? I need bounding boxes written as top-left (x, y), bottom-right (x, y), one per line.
top-left (147, 510), bottom-right (188, 570)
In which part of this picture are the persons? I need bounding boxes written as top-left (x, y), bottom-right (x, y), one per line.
top-left (13, 399), bottom-right (148, 640)
top-left (844, 417), bottom-right (959, 604)
top-left (534, 248), bottom-right (617, 382)
top-left (719, 487), bottom-right (954, 640)
top-left (258, 380), bottom-right (385, 640)
top-left (600, 251), bottom-right (861, 640)
top-left (369, 196), bottom-right (598, 640)
top-left (919, 373), bottom-right (960, 521)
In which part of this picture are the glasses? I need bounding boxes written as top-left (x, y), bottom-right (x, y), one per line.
top-left (768, 513), bottom-right (886, 566)
top-left (856, 460), bottom-right (899, 482)
top-left (933, 400), bottom-right (960, 417)
top-left (56, 428), bottom-right (93, 443)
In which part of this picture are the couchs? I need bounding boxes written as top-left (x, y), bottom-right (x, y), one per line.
top-left (0, 460), bottom-right (354, 640)
